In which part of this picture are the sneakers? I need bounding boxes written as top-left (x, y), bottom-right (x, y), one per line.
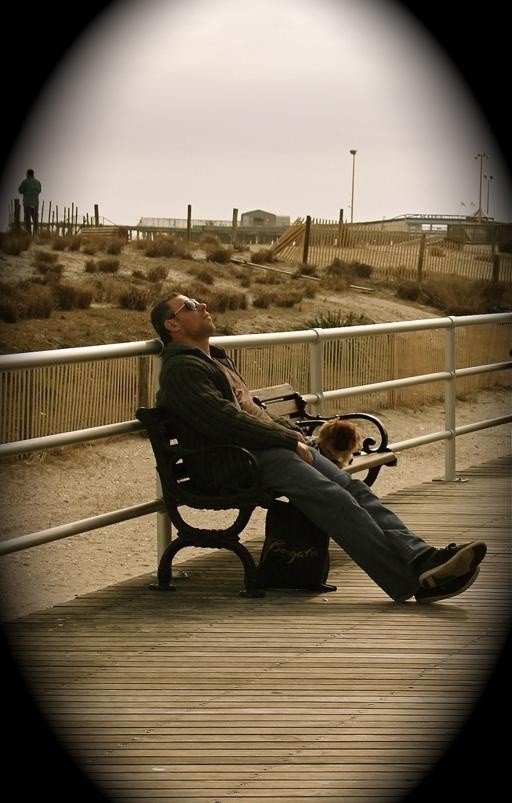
top-left (415, 539), bottom-right (487, 605)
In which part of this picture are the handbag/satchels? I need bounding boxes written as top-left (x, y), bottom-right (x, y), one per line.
top-left (256, 501), bottom-right (336, 592)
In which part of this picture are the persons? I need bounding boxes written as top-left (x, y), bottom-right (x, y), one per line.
top-left (15, 165), bottom-right (43, 231)
top-left (149, 289), bottom-right (488, 606)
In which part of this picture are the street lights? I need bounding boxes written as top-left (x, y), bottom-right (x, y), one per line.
top-left (475, 152), bottom-right (493, 223)
top-left (350, 149), bottom-right (356, 223)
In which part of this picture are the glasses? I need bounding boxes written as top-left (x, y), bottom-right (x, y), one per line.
top-left (169, 298), bottom-right (198, 319)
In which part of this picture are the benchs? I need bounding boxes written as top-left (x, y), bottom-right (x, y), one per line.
top-left (136, 383), bottom-right (398, 598)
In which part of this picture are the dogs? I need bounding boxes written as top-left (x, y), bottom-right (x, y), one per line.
top-left (308, 419), bottom-right (360, 466)
